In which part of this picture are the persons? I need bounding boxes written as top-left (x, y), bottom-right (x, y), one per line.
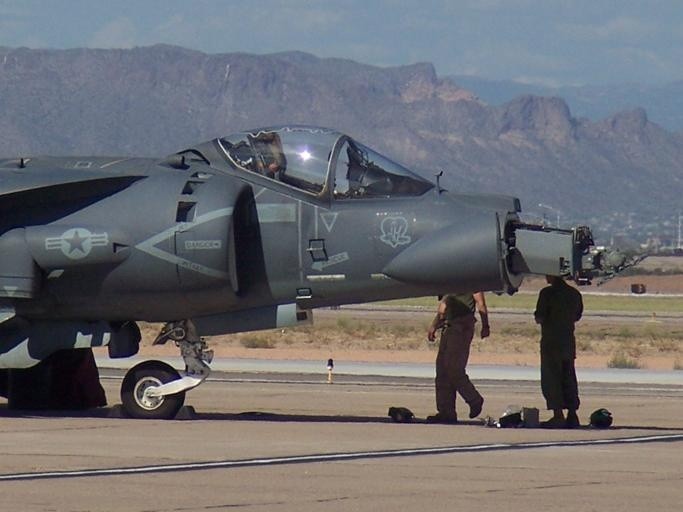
top-left (428, 291), bottom-right (490, 423)
top-left (534, 275), bottom-right (583, 427)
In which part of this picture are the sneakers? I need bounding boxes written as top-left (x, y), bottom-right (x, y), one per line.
top-left (540, 416), bottom-right (564, 428)
top-left (562, 414), bottom-right (579, 428)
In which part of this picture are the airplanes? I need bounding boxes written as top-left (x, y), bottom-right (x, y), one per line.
top-left (0, 123), bottom-right (649, 419)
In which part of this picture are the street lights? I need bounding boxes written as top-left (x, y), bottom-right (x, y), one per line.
top-left (537, 201), bottom-right (560, 228)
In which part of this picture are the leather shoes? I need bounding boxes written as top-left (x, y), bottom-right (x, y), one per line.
top-left (426, 414), bottom-right (456, 422)
top-left (469, 397), bottom-right (484, 418)
